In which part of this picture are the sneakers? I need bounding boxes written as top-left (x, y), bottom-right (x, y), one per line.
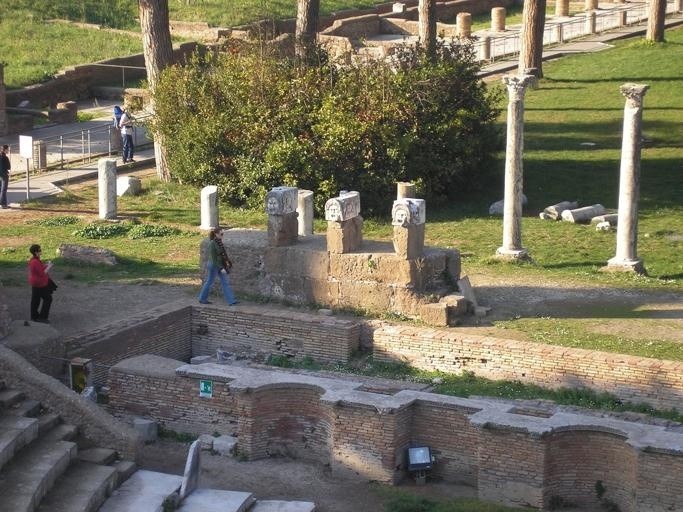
top-left (198, 300), bottom-right (212, 304)
top-left (228, 301), bottom-right (241, 305)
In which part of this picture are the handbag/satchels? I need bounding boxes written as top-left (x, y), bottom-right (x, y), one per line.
top-left (48, 278), bottom-right (58, 294)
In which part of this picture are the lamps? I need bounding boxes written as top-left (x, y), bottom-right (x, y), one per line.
top-left (407, 445), bottom-right (438, 483)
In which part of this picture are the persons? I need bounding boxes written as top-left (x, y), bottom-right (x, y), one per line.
top-left (0, 144), bottom-right (11, 209)
top-left (119, 106), bottom-right (136, 163)
top-left (199, 229), bottom-right (240, 306)
top-left (29, 245), bottom-right (55, 323)
